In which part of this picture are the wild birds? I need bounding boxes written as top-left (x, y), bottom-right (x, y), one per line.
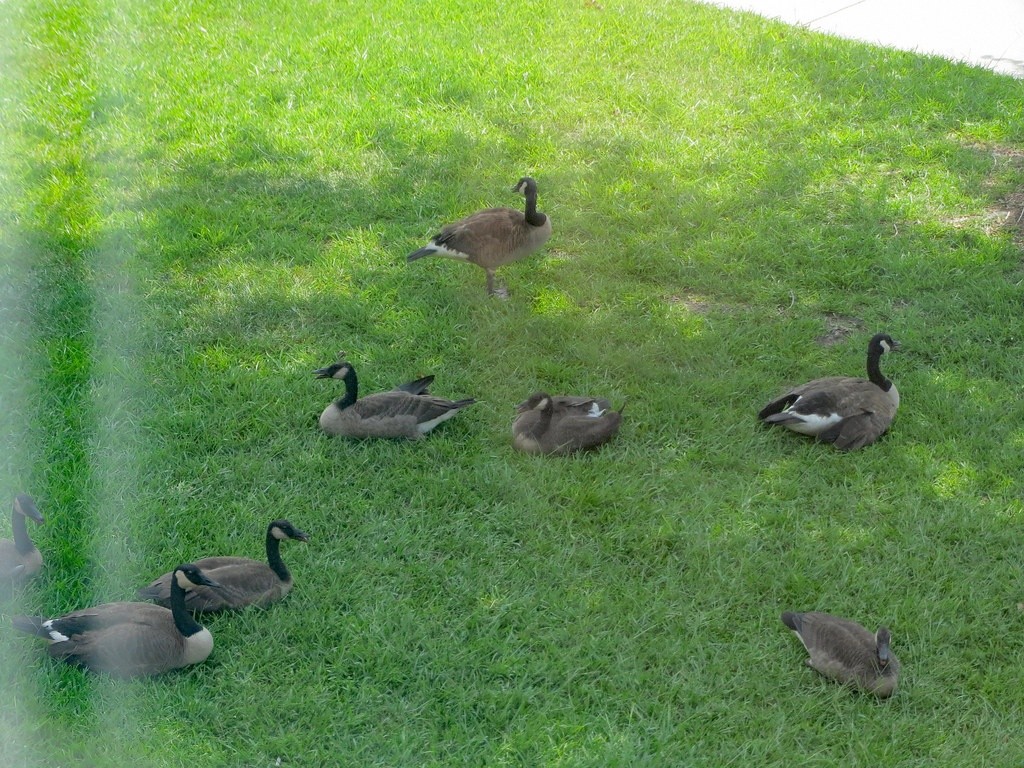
top-left (312, 362), bottom-right (478, 439)
top-left (512, 389), bottom-right (622, 456)
top-left (409, 177), bottom-right (551, 298)
top-left (0, 493), bottom-right (310, 678)
top-left (758, 332), bottom-right (904, 453)
top-left (782, 611), bottom-right (902, 699)
